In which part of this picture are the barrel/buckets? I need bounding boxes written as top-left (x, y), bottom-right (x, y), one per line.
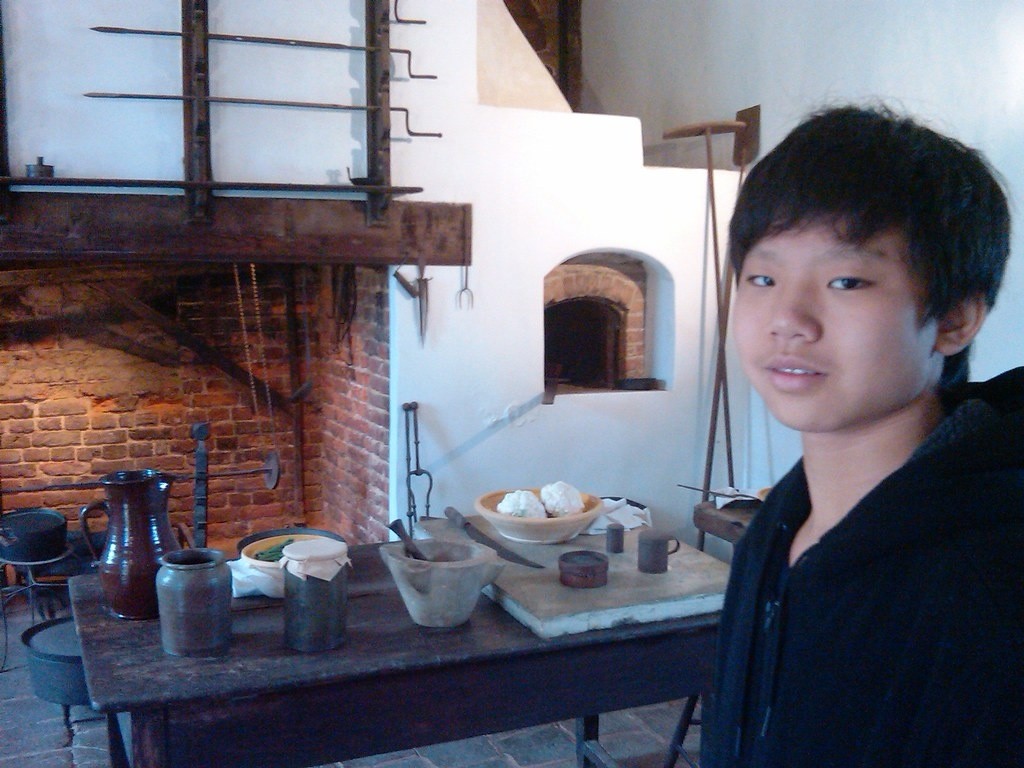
top-left (17, 617), bottom-right (88, 706)
top-left (0, 508), bottom-right (65, 562)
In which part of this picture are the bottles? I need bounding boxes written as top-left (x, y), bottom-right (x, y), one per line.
top-left (155, 547), bottom-right (231, 656)
top-left (607, 522), bottom-right (623, 552)
top-left (281, 540), bottom-right (350, 652)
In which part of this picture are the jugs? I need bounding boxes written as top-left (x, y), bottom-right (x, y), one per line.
top-left (79, 468), bottom-right (183, 621)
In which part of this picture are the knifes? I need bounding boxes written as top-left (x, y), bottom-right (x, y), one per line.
top-left (444, 506), bottom-right (545, 569)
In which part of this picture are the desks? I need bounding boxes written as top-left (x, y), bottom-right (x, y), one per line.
top-left (71, 539), bottom-right (721, 768)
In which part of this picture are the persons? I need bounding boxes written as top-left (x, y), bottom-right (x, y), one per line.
top-left (699, 106), bottom-right (1024, 768)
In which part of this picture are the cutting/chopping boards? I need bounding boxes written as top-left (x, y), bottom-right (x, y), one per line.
top-left (422, 515), bottom-right (733, 640)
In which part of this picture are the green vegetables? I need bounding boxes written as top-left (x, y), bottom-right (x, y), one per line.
top-left (255, 536), bottom-right (295, 563)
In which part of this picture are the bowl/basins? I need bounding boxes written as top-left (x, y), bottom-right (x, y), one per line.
top-left (236, 527), bottom-right (347, 582)
top-left (475, 487), bottom-right (603, 544)
top-left (561, 550), bottom-right (611, 589)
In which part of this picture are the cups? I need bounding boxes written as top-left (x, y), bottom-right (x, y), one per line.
top-left (638, 527), bottom-right (679, 575)
